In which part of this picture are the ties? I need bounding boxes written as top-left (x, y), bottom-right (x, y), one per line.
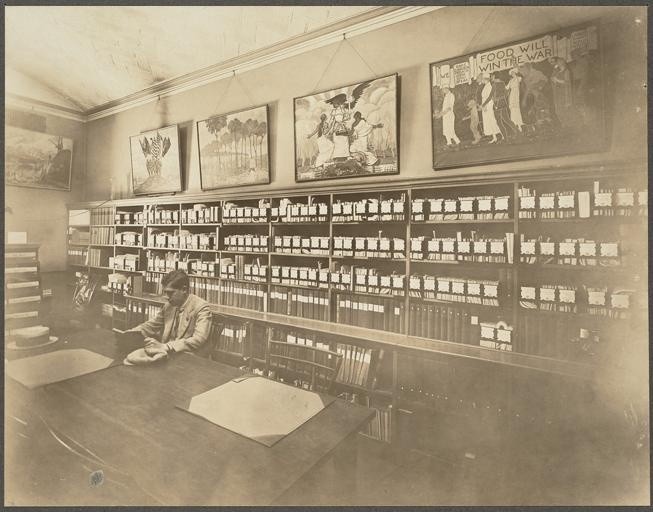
top-left (173, 309), bottom-right (179, 339)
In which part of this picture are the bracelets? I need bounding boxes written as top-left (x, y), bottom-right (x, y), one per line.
top-left (164, 343), bottom-right (170, 353)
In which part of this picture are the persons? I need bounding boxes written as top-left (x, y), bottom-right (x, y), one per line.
top-left (433, 50), bottom-right (592, 154)
top-left (306, 110), bottom-right (337, 173)
top-left (112, 269), bottom-right (213, 361)
top-left (347, 111), bottom-right (384, 166)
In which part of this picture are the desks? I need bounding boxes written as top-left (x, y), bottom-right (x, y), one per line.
top-left (5, 327), bottom-right (376, 508)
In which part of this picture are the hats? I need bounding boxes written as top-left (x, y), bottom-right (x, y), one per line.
top-left (123, 348), bottom-right (168, 366)
top-left (481, 72), bottom-right (491, 79)
top-left (6, 326), bottom-right (58, 349)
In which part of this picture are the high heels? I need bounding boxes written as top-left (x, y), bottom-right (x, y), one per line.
top-left (488, 138), bottom-right (497, 144)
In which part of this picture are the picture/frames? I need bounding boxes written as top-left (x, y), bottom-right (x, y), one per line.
top-left (5, 125), bottom-right (73, 192)
top-left (293, 72), bottom-right (400, 183)
top-left (129, 123), bottom-right (184, 196)
top-left (196, 103), bottom-right (271, 191)
top-left (428, 17), bottom-right (608, 171)
